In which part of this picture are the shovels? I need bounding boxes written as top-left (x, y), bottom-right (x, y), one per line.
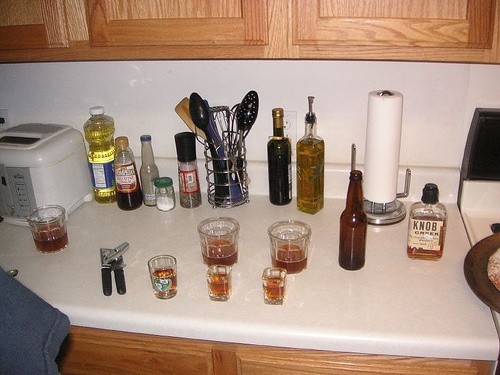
top-left (174, 97), bottom-right (205, 141)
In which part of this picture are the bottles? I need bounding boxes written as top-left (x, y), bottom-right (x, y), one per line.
top-left (174, 131), bottom-right (202, 209)
top-left (266, 107), bottom-right (293, 206)
top-left (153, 177), bottom-right (176, 211)
top-left (338, 170), bottom-right (368, 271)
top-left (138, 134), bottom-right (159, 206)
top-left (295, 95), bottom-right (325, 215)
top-left (83, 105), bottom-right (117, 204)
top-left (406, 182), bottom-right (447, 260)
top-left (112, 136), bottom-right (144, 211)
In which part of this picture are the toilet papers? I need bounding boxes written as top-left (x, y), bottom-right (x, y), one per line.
top-left (359, 89), bottom-right (404, 205)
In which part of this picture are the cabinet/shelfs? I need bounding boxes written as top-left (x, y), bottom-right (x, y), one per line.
top-left (54, 326), bottom-right (491, 375)
top-left (0, 0), bottom-right (499, 65)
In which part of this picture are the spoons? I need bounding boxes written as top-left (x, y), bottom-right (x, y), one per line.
top-left (235, 90), bottom-right (259, 188)
top-left (188, 91), bottom-right (226, 206)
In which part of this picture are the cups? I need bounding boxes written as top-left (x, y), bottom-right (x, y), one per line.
top-left (261, 266), bottom-right (288, 305)
top-left (147, 255), bottom-right (178, 299)
top-left (206, 264), bottom-right (232, 301)
top-left (267, 220), bottom-right (311, 273)
top-left (26, 204), bottom-right (69, 255)
top-left (197, 216), bottom-right (238, 268)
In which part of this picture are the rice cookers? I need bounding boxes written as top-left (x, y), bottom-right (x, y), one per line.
top-left (0, 122), bottom-right (95, 228)
